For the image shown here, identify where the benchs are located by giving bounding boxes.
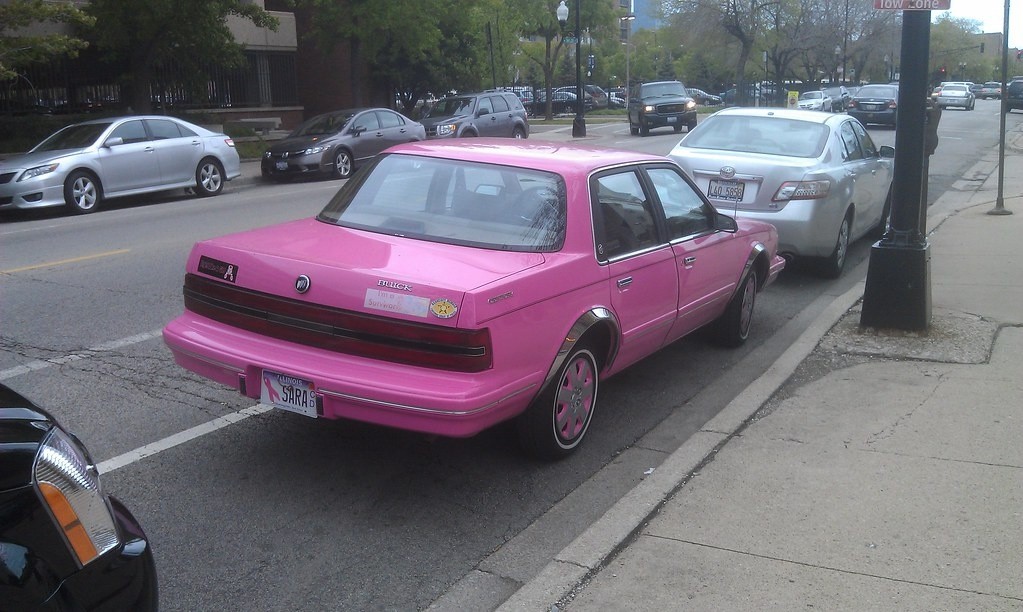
[353,204,557,242]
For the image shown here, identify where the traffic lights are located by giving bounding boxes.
[1017,50,1021,59]
[941,66,946,82]
[980,42,984,53]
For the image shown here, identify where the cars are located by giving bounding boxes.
[664,105,897,280]
[719,81,790,105]
[928,81,984,106]
[937,84,976,111]
[1006,75,1023,91]
[0,383,160,612]
[160,137,786,457]
[0,114,242,218]
[846,80,900,99]
[394,84,628,116]
[846,83,899,127]
[260,107,427,183]
[794,91,833,112]
[821,86,853,112]
[685,88,723,106]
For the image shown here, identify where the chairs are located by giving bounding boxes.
[454,189,500,220]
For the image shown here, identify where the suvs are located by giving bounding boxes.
[1005,80,1023,112]
[628,79,700,137]
[418,92,530,140]
[982,82,1001,100]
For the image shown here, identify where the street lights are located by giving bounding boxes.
[620,11,636,109]
[556,0,588,137]
